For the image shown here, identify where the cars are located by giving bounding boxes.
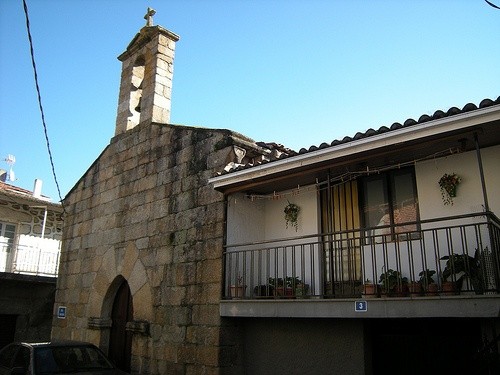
[1,339,127,375]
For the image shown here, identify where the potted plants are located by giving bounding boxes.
[231,275,247,300]
[283,201,300,233]
[362,248,487,296]
[254,275,309,298]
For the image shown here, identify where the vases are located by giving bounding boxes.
[444,185,456,198]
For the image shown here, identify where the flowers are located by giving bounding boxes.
[439,173,462,206]
